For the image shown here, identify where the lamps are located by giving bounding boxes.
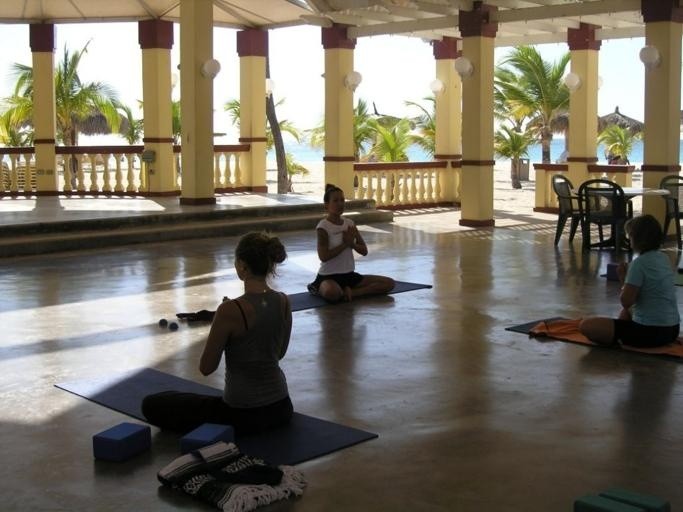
[430,80,444,95]
[639,46,661,71]
[597,74,603,91]
[565,72,581,92]
[344,71,362,92]
[266,78,275,98]
[200,58,221,80]
[454,57,473,77]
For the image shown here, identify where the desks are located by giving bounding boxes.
[576,188,670,256]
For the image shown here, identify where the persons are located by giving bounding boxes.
[140,233,293,441]
[577,215,680,348]
[308,183,395,303]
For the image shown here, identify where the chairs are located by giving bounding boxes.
[551,174,604,249]
[658,174,683,248]
[578,180,633,256]
